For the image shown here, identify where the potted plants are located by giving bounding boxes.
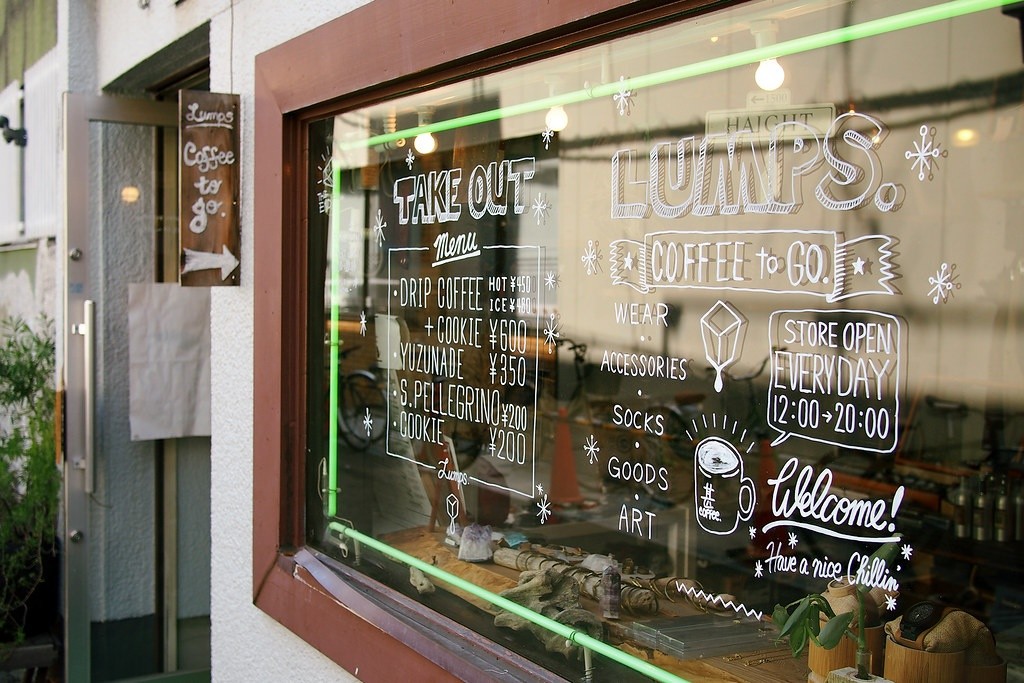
[775,535,895,683]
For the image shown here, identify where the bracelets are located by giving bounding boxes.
[515,540,737,618]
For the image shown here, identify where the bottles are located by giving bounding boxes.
[1012,487,1024,540]
[973,481,992,540]
[602,552,621,619]
[994,475,1011,541]
[954,474,971,537]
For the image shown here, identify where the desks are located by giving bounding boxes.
[504,497,755,580]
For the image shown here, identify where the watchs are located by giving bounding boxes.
[899,595,945,642]
